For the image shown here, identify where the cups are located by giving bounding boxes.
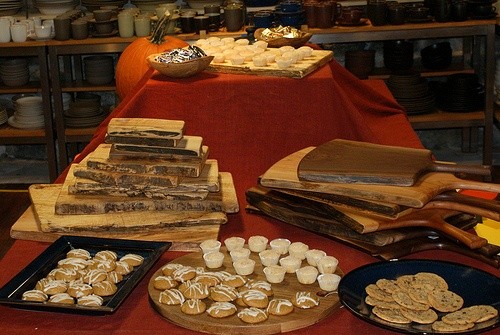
[0,0,498,43]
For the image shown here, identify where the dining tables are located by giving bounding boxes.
[0,43,499,334]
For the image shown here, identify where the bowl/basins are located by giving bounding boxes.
[146,51,216,78]
[383,39,455,75]
[83,55,116,86]
[1,59,31,89]
[254,26,314,47]
[344,49,375,81]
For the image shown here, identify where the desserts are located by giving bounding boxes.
[191,37,313,69]
[200,236,341,291]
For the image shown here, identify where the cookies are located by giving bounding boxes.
[154,264,320,323]
[23,249,144,307]
[365,272,498,332]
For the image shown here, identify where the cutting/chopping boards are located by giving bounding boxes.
[244,139,500,262]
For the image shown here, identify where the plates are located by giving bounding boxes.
[0,92,109,128]
[338,258,500,335]
[386,77,486,115]
[0,234,173,316]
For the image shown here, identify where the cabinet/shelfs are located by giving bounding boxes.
[0,1,500,193]
